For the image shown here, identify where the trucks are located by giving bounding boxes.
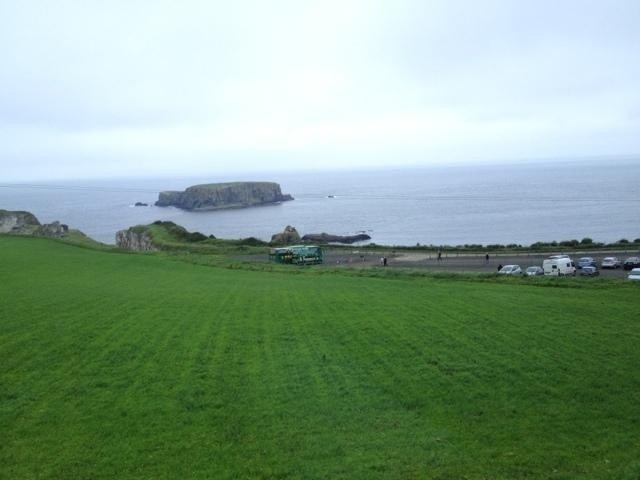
[542,255,577,278]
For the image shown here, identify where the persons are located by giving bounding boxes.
[438,250,442,261]
[498,265,503,271]
[380,257,387,266]
[486,252,489,264]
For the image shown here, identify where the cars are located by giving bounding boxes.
[577,256,640,281]
[497,265,543,276]
